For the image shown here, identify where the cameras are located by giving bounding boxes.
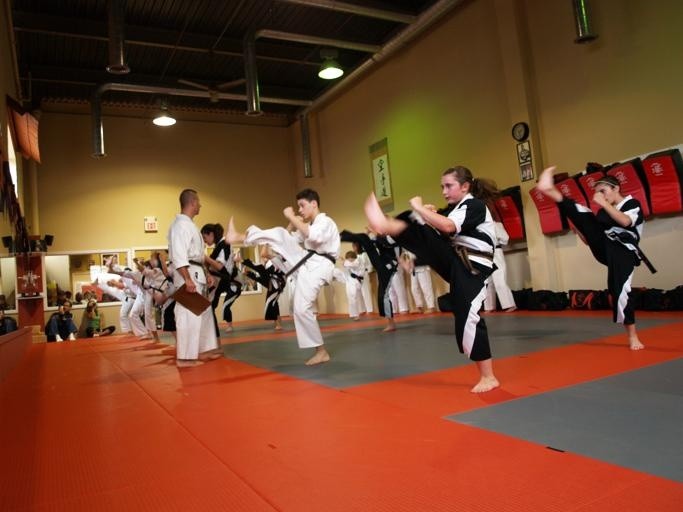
[89,303,96,307]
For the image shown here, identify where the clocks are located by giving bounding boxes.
[511,122,532,144]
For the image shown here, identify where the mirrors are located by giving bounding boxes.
[0,160,36,345]
[40,243,131,312]
[132,244,264,300]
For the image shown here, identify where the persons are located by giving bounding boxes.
[536,165,658,351]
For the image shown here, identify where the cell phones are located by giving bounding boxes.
[59,305,64,314]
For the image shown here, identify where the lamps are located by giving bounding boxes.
[151,95,178,129]
[317,46,347,84]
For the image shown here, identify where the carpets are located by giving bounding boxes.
[200,310,680,393]
[440,354,682,484]
[156,316,390,347]
